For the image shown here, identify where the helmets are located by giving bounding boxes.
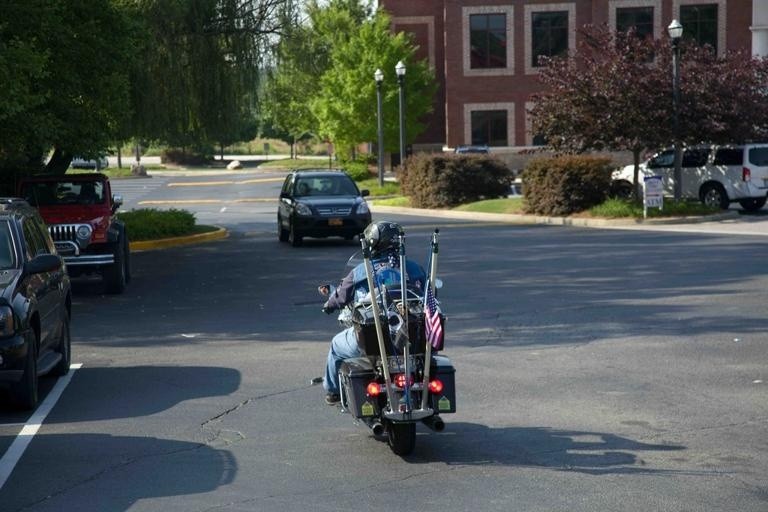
[364,221,405,256]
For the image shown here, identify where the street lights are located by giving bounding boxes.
[373,68,385,188]
[668,19,684,207]
[394,59,407,164]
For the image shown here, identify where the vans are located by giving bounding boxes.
[610,142,767,212]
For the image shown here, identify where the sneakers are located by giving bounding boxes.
[326,392,340,404]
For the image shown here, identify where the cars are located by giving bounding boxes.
[71,151,109,170]
[277,167,371,247]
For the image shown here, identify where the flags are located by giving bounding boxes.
[379,283,405,349]
[422,280,444,351]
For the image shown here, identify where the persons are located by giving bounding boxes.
[321,220,441,408]
[297,182,310,196]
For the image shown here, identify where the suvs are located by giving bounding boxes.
[14,172,132,293]
[0,195,74,411]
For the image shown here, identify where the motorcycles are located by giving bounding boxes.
[308,249,457,461]
